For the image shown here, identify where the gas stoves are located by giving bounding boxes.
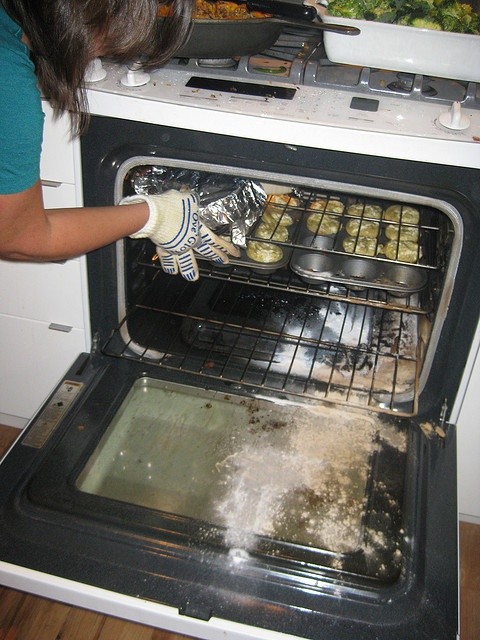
[51,30,479,143]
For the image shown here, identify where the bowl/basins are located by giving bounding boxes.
[320,15,480,82]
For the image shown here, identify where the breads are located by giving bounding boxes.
[346,203,382,220]
[308,200,344,218]
[342,236,379,257]
[269,194,300,209]
[246,242,284,263]
[384,239,422,262]
[304,211,343,235]
[255,221,289,242]
[327,0,480,35]
[260,209,294,227]
[384,222,422,241]
[384,204,420,225]
[345,219,380,237]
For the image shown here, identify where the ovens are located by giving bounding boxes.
[2,93,479,638]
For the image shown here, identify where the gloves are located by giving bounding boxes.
[119,186,242,282]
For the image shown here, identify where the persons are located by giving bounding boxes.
[1,0,243,285]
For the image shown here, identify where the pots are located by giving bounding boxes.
[145,16,358,61]
